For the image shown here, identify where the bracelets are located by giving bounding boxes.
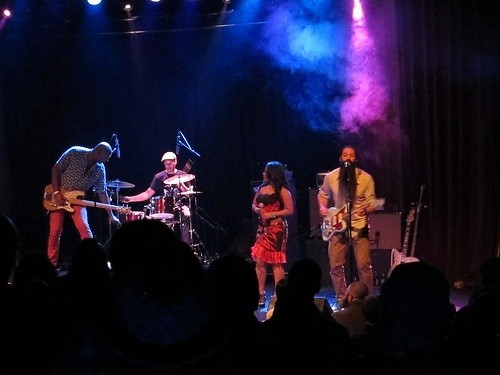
[52,190,59,196]
[271,212,277,218]
[255,208,258,213]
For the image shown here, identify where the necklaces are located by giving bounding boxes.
[267,184,274,195]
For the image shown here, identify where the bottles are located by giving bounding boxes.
[381,272,386,284]
[374,271,379,285]
[284,165,288,178]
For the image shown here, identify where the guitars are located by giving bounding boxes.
[43,184,131,215]
[319,199,385,241]
[387,209,419,279]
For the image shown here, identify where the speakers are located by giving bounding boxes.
[366,211,404,253]
[309,188,335,237]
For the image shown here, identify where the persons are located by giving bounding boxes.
[251,161,295,306]
[0,214,500,375]
[317,146,380,306]
[48,141,121,269]
[123,151,193,244]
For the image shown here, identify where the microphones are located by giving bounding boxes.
[375,231,380,239]
[115,139,120,158]
[175,135,182,154]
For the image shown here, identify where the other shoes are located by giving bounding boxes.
[56,265,61,271]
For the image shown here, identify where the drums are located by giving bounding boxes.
[145,205,179,225]
[151,196,175,220]
[119,211,144,224]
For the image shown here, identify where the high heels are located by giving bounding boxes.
[257,291,267,305]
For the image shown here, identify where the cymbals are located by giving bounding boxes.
[163,174,195,184]
[107,181,135,189]
[177,191,203,198]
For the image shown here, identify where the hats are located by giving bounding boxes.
[161,152,176,162]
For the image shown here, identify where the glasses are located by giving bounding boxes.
[164,162,175,165]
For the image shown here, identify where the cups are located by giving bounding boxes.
[288,171,292,178]
